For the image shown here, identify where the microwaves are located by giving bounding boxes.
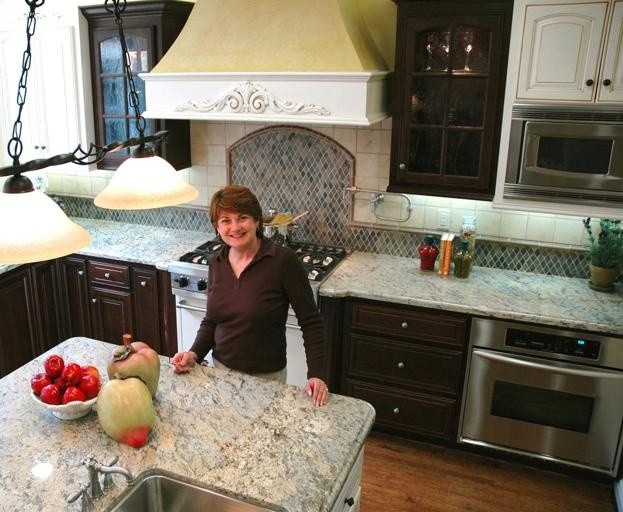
[502,104,623,208]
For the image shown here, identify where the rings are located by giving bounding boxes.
[325,389,328,391]
[320,388,323,391]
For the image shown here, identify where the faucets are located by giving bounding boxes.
[81,452,136,500]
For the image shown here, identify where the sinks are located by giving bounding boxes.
[104,467,287,512]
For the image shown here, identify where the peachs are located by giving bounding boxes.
[97,373,157,449]
[107,333,161,399]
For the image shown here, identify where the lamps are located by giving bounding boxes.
[94,12,198,211]
[1,11,92,265]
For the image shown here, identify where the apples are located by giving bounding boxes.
[31,373,52,395]
[61,363,83,386]
[81,365,99,381]
[41,384,61,404]
[43,355,64,377]
[63,387,85,404]
[80,376,101,398]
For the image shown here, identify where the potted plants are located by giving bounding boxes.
[582,213,623,293]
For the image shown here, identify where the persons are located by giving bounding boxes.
[171,185,329,406]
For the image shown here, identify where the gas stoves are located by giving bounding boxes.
[171,235,345,309]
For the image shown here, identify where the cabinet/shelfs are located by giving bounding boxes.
[55,214,132,343]
[329,443,363,512]
[83,1,194,175]
[511,0,623,109]
[134,221,176,354]
[0,261,54,375]
[321,253,469,452]
[389,0,511,200]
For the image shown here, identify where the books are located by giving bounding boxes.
[439,233,456,276]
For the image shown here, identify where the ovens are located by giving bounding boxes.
[456,316,623,479]
